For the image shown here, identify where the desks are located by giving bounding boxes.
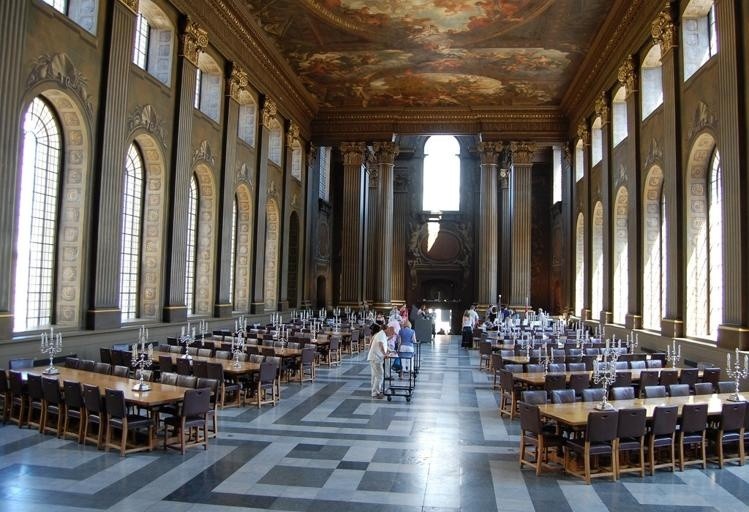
[501,355,651,364]
[489,336,594,344]
[5,365,195,450]
[512,327,552,329]
[232,333,330,367]
[513,367,704,391]
[536,392,749,474]
[124,350,260,407]
[194,339,302,385]
[300,329,352,355]
[492,344,580,353]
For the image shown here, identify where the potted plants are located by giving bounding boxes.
[304,310,305,317]
[582,323,584,331]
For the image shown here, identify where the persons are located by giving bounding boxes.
[469,302,514,322]
[373,303,428,334]
[461,309,474,351]
[397,319,417,381]
[366,325,396,399]
[385,326,399,381]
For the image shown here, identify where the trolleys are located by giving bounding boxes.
[396,339,421,379]
[415,316,433,344]
[383,351,416,403]
[424,314,437,338]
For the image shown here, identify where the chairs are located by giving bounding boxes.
[204,342,220,358]
[114,366,129,378]
[582,389,604,402]
[716,382,736,393]
[113,344,129,351]
[315,331,323,334]
[353,324,360,328]
[170,346,184,354]
[479,342,492,371]
[357,326,371,351]
[292,328,300,336]
[564,343,576,349]
[270,330,278,334]
[122,350,135,371]
[274,341,287,348]
[179,340,187,346]
[695,402,746,469]
[216,350,231,360]
[514,344,527,355]
[317,334,329,340]
[282,342,300,377]
[208,363,241,410]
[679,368,699,394]
[629,361,645,369]
[658,371,678,392]
[261,330,269,334]
[530,343,542,349]
[668,384,689,397]
[159,344,170,352]
[555,339,567,344]
[167,337,178,346]
[580,335,590,338]
[304,332,314,339]
[65,357,79,369]
[247,333,257,338]
[137,352,160,381]
[239,354,265,398]
[246,325,255,333]
[518,350,527,356]
[190,341,204,349]
[658,403,708,472]
[521,390,547,405]
[500,350,514,357]
[618,355,633,361]
[632,354,646,361]
[193,360,208,378]
[611,387,634,400]
[213,335,223,341]
[316,334,329,340]
[213,331,222,335]
[587,326,592,332]
[550,349,565,356]
[221,343,232,351]
[526,364,544,373]
[517,401,568,476]
[234,333,244,338]
[591,373,610,391]
[567,362,586,371]
[584,348,600,355]
[646,359,661,368]
[621,343,631,354]
[281,331,292,336]
[286,349,315,385]
[565,339,576,344]
[155,375,198,437]
[111,349,122,365]
[600,408,647,480]
[484,339,497,344]
[100,347,112,364]
[551,390,576,403]
[267,327,276,331]
[298,338,320,368]
[250,329,261,334]
[515,339,527,344]
[224,352,248,396]
[613,348,627,354]
[189,378,220,443]
[568,374,589,397]
[569,349,582,356]
[544,375,566,399]
[247,346,259,355]
[246,338,258,345]
[188,347,198,356]
[95,363,111,375]
[263,334,273,340]
[79,360,95,372]
[176,358,193,376]
[598,363,610,370]
[222,331,232,336]
[580,343,591,349]
[625,405,679,476]
[234,345,242,352]
[600,348,615,354]
[137,372,178,431]
[565,411,618,485]
[581,356,597,371]
[549,335,560,339]
[473,328,483,347]
[288,337,298,343]
[304,333,316,339]
[83,384,107,450]
[42,377,65,439]
[242,362,277,408]
[492,354,506,391]
[63,380,86,444]
[499,370,521,420]
[732,402,749,462]
[343,330,359,356]
[204,333,211,338]
[602,355,617,362]
[579,339,590,343]
[519,335,527,340]
[126,369,154,414]
[530,349,545,356]
[317,335,341,363]
[610,372,631,387]
[500,339,513,344]
[198,349,212,357]
[694,383,712,395]
[593,339,601,343]
[548,356,565,364]
[530,339,541,344]
[27,373,45,433]
[7,370,29,429]
[584,326,587,331]
[547,343,558,350]
[302,329,310,333]
[159,356,177,377]
[246,357,282,404]
[0,369,11,425]
[548,363,566,372]
[541,339,552,344]
[504,364,523,373]
[698,368,721,394]
[194,334,202,340]
[235,337,245,344]
[224,336,232,342]
[533,336,543,340]
[644,386,665,398]
[480,333,497,344]
[287,343,317,379]
[164,388,210,455]
[9,359,33,370]
[632,371,659,399]
[609,342,617,348]
[529,357,546,364]
[341,324,350,328]
[504,336,514,340]
[651,353,665,368]
[262,348,275,357]
[593,343,606,348]
[256,326,266,330]
[492,326,501,331]
[565,355,581,363]
[317,336,338,367]
[294,332,303,337]
[568,335,576,340]
[294,332,304,337]
[262,340,274,346]
[105,387,155,457]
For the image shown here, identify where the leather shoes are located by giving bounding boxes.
[373,395,384,400]
[384,377,394,381]
[378,394,384,397]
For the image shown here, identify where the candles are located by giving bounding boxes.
[336,307,338,312]
[585,331,588,338]
[203,319,204,326]
[276,313,278,321]
[301,313,303,320]
[206,322,208,331]
[339,318,341,324]
[192,328,193,336]
[139,327,141,339]
[612,334,615,342]
[55,334,58,345]
[678,345,680,354]
[636,334,639,342]
[293,309,295,316]
[518,328,519,335]
[286,329,288,339]
[513,326,515,333]
[352,313,353,319]
[310,323,312,329]
[603,327,605,333]
[59,333,62,343]
[727,354,730,368]
[142,324,144,338]
[338,309,341,313]
[744,355,748,369]
[668,345,669,354]
[244,318,247,329]
[349,315,350,321]
[235,322,237,332]
[355,315,356,321]
[150,343,153,355]
[596,362,599,374]
[322,308,324,314]
[309,309,311,314]
[312,310,314,315]
[237,334,240,347]
[290,312,292,318]
[232,337,234,348]
[606,340,609,349]
[558,320,559,329]
[735,348,738,360]
[595,326,597,334]
[333,317,334,323]
[282,325,284,336]
[345,308,346,312]
[280,316,282,323]
[626,335,629,344]
[242,338,244,349]
[347,306,348,311]
[146,328,148,340]
[553,323,556,332]
[193,327,195,336]
[593,360,596,374]
[324,309,327,315]
[612,362,615,374]
[41,333,44,344]
[610,361,612,375]
[199,321,201,328]
[135,343,137,356]
[148,345,151,357]
[45,332,47,343]
[509,328,512,335]
[318,322,319,329]
[333,308,335,314]
[314,322,315,327]
[319,310,321,316]
[182,327,183,335]
[618,339,621,349]
[349,307,350,312]
[296,311,297,317]
[239,317,241,324]
[604,355,606,365]
[272,316,274,323]
[132,344,135,358]
[188,322,190,333]
[242,314,244,323]
[560,324,564,331]
[576,330,580,338]
[183,327,185,334]
[672,340,675,352]
[307,312,308,318]
[631,331,633,342]
[278,328,280,335]
[50,327,54,338]
[598,324,601,332]
